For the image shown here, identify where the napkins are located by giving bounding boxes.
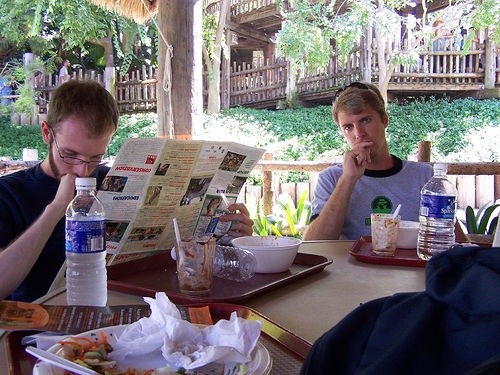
[113,291,265,371]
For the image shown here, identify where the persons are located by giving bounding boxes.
[33,70,42,89]
[0,69,13,107]
[302,80,471,246]
[427,26,488,74]
[58,59,70,86]
[0,80,254,304]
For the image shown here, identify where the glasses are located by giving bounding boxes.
[49,126,110,166]
[333,81,369,103]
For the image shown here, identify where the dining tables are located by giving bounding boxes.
[43,239,424,343]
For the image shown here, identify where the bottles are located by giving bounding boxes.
[170,245,257,282]
[416,164,458,260]
[65,178,108,307]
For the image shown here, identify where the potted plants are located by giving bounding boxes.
[461,202,500,244]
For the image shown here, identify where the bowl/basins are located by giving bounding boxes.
[396,220,420,249]
[231,235,302,274]
[32,323,273,375]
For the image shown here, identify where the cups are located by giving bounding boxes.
[175,235,216,295]
[371,213,401,256]
